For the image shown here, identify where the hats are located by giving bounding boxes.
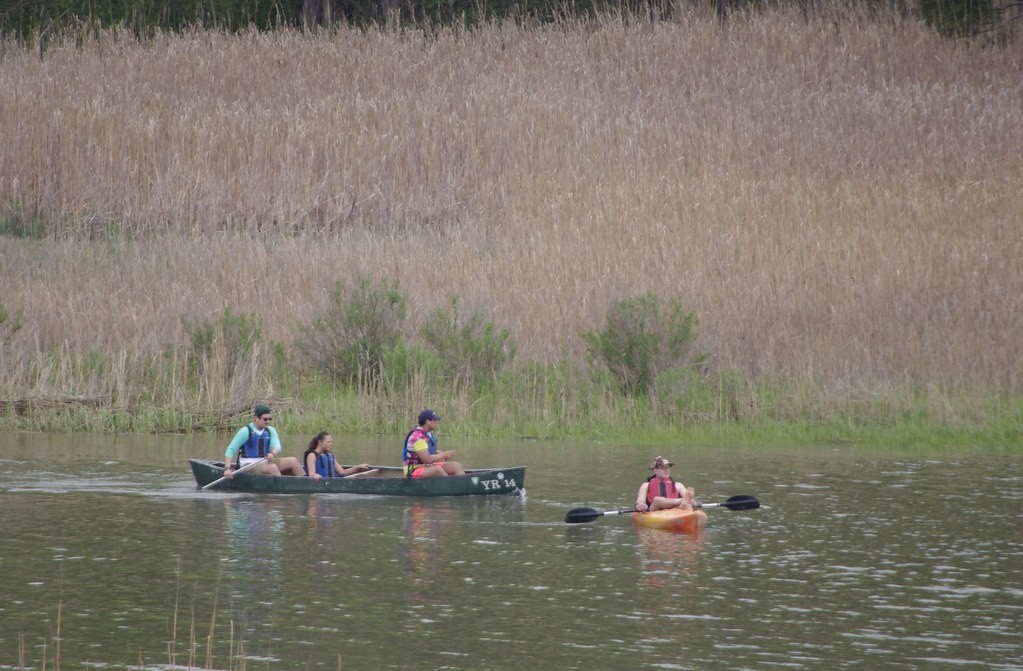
[650,456,674,470]
[255,406,270,415]
[420,409,441,421]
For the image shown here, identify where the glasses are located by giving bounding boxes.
[264,418,271,421]
[654,465,670,470]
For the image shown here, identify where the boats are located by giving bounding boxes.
[189,457,528,497]
[631,509,709,537]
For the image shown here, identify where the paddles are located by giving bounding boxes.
[564,494,760,523]
[195,456,268,490]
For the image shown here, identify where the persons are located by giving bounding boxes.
[224,405,306,481]
[636,456,696,510]
[402,409,464,477]
[303,431,370,479]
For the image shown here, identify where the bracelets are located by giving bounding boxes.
[271,452,275,457]
